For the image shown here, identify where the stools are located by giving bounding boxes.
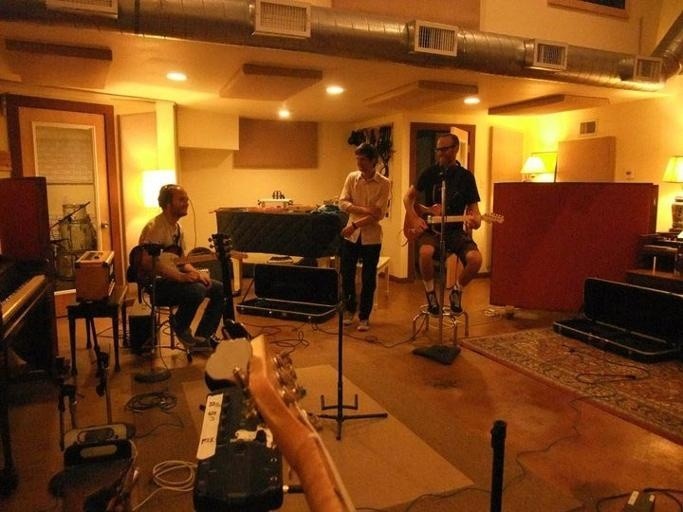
[154,305,192,363]
[356,257,390,308]
[413,249,468,346]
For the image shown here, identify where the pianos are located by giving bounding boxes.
[1,259,55,349]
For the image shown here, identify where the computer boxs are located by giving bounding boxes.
[128,296,160,354]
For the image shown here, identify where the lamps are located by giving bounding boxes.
[662,156,683,232]
[520,154,547,182]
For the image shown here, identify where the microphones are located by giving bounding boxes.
[439,157,446,177]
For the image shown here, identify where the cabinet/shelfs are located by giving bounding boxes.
[624,233,682,295]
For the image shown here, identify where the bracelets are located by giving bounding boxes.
[352,221,358,230]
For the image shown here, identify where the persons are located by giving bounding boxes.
[137,184,225,352]
[337,142,392,331]
[403,132,483,316]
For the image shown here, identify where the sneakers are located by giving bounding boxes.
[425,289,440,317]
[189,335,219,353]
[448,287,464,315]
[356,319,370,331]
[167,314,196,346]
[342,311,353,326]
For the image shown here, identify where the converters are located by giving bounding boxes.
[625,489,656,512]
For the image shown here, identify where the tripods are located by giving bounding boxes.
[305,300,387,439]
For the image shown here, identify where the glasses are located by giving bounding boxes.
[433,145,458,153]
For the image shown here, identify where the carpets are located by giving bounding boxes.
[461,326,682,445]
[182,364,474,510]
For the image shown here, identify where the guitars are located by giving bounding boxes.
[128,243,248,281]
[210,234,251,341]
[403,202,504,234]
[234,334,357,507]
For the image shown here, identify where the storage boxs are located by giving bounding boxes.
[73,250,116,303]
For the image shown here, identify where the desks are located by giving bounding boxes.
[65,285,129,371]
[215,208,348,292]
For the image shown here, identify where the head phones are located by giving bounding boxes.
[163,185,172,204]
